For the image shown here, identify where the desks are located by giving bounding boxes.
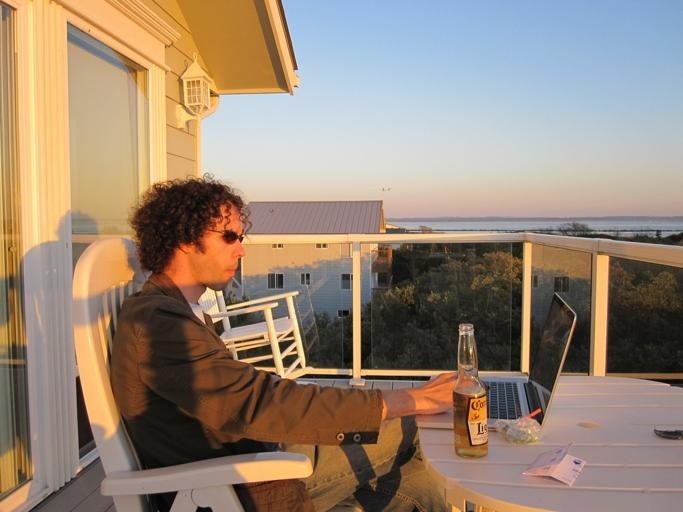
[416,372,682,512]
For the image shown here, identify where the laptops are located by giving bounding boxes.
[414,293,577,431]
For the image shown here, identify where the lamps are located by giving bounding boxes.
[179,52,215,117]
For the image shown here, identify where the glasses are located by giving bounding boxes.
[208,229,243,245]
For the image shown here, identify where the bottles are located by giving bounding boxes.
[452,324,488,458]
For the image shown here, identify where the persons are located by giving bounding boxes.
[110,176,490,512]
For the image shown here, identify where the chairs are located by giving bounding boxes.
[70,232,314,512]
